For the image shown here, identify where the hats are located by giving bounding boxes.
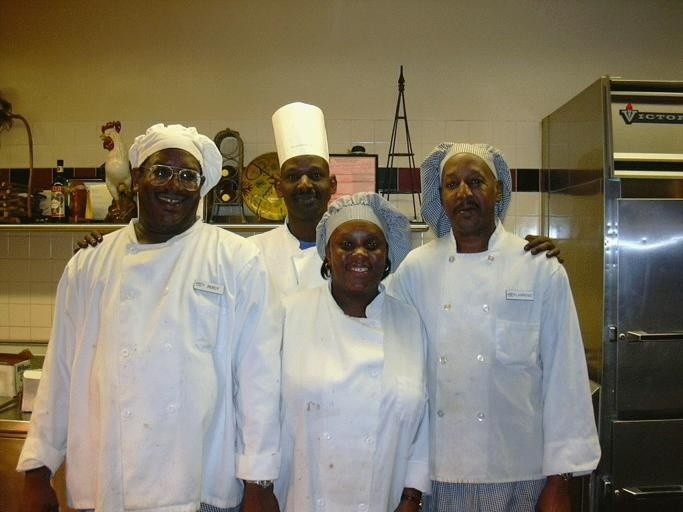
[271,102,330,167]
[128,123,222,196]
[420,141,512,239]
[316,192,413,273]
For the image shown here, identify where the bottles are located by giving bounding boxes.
[50,160,68,223]
[222,165,235,178]
[219,190,237,202]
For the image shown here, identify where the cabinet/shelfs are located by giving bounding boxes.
[0,420,67,512]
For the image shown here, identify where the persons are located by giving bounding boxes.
[387,140,603,512]
[16,123,278,512]
[69,101,564,512]
[268,192,433,512]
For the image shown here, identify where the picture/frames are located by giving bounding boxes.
[329,154,378,193]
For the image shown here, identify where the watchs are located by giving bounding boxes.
[400,494,425,508]
[556,472,575,481]
[245,479,275,489]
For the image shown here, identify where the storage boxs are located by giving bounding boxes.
[22,369,43,412]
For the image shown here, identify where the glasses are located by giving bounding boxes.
[148,165,201,192]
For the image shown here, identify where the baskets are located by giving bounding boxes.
[0,108,46,226]
